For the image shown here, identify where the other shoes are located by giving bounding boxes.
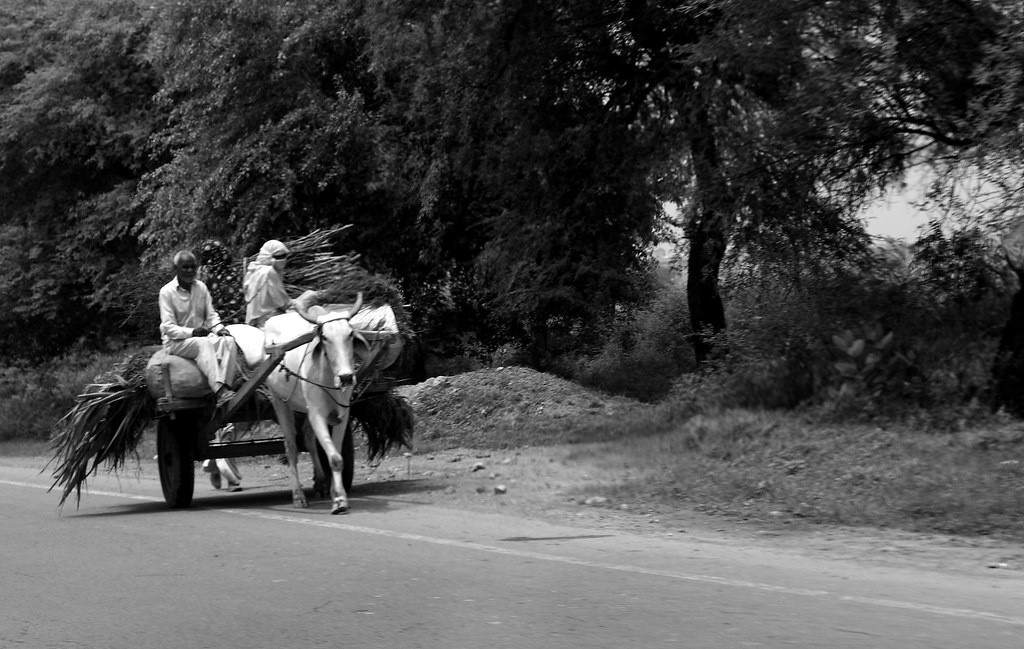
[216,392,236,408]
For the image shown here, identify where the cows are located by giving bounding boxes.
[265,291,372,515]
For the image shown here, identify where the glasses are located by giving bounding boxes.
[182,264,198,269]
[273,253,287,259]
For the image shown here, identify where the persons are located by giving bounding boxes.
[160,251,243,491]
[244,241,296,328]
